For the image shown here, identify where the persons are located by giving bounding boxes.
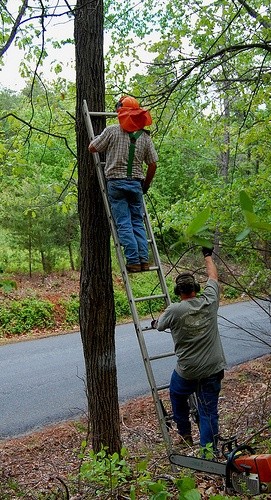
[89,95,159,272]
[151,246,227,459]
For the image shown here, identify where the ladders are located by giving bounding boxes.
[79,97,219,486]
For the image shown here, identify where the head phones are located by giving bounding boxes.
[115,97,126,110]
[174,273,200,295]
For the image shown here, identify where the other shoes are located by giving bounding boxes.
[126,263,149,272]
[174,435,193,447]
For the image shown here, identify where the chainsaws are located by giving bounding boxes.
[167,448,271,496]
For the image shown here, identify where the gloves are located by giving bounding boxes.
[202,246,213,256]
[151,320,156,328]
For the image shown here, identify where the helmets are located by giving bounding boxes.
[116,95,140,108]
